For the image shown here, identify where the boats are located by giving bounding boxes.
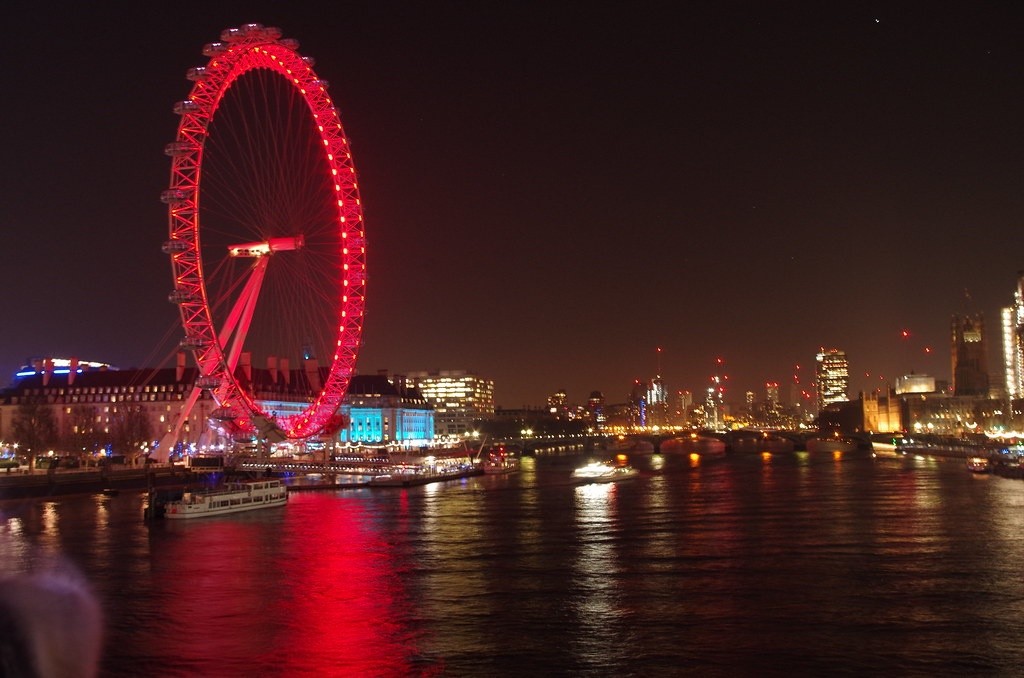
[161,478,290,518]
[484,447,519,474]
